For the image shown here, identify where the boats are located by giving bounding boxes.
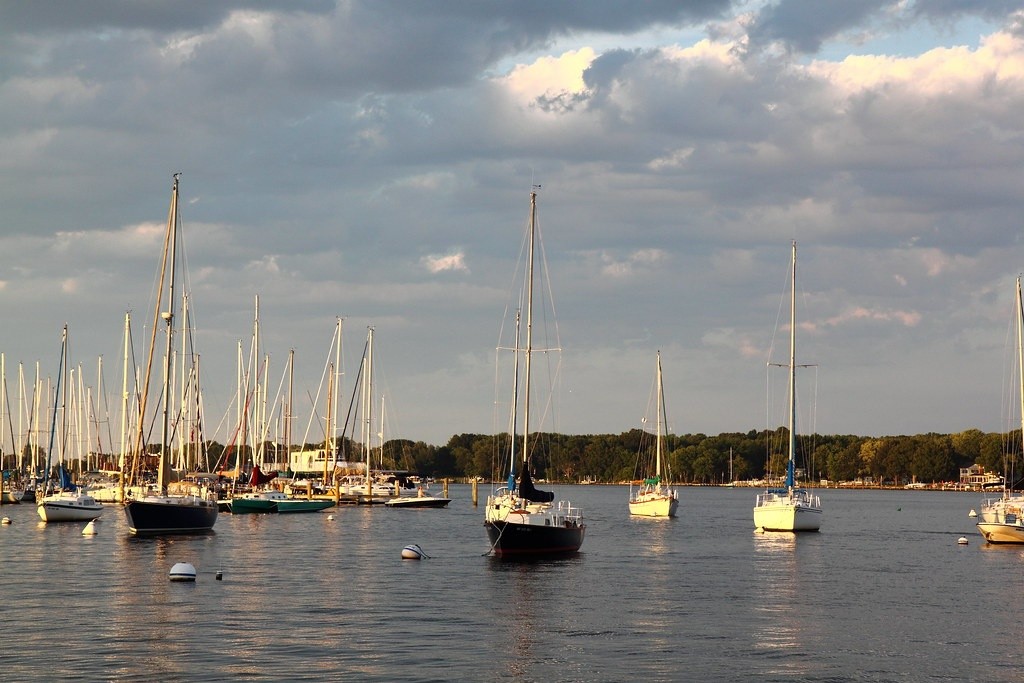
[630,349,679,519]
[483,170,587,562]
[974,276,1024,544]
[753,238,823,532]
[0,169,455,536]
